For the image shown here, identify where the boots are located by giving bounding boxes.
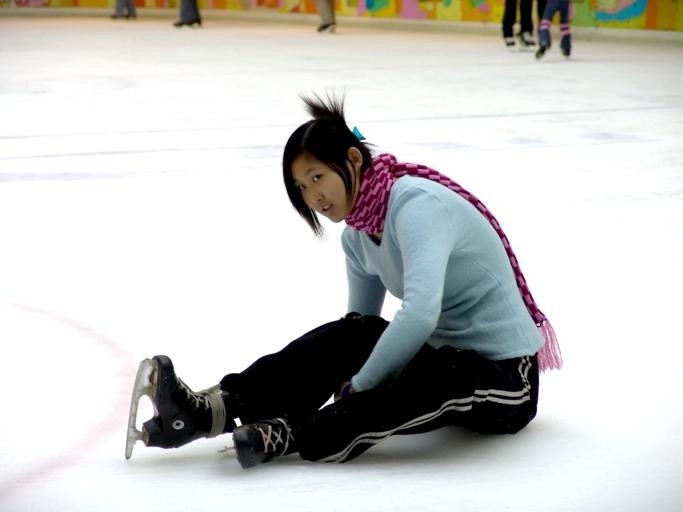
[233,416,299,468]
[141,355,236,448]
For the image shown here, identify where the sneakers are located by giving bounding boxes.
[536,45,569,57]
[174,17,200,25]
[112,14,135,18]
[318,21,334,32]
[505,32,535,45]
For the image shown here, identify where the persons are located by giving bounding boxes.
[531,1,571,59]
[139,90,563,471]
[312,1,337,34]
[171,0,202,27]
[109,1,137,19]
[502,1,537,48]
